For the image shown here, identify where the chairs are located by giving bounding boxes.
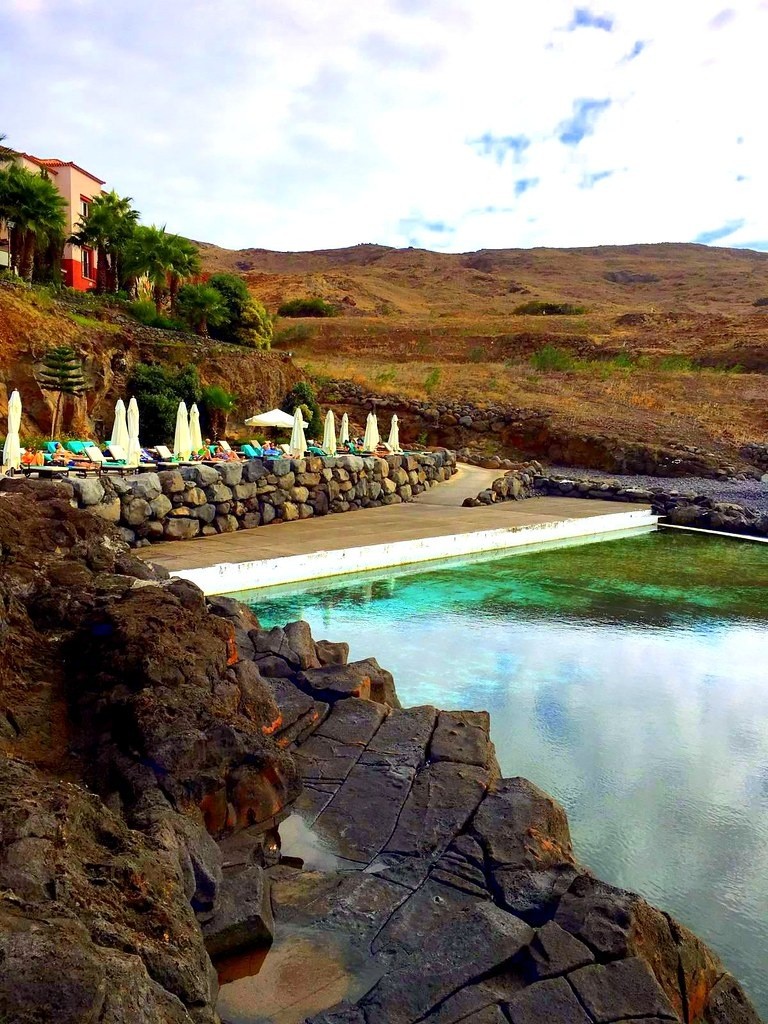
[43,441,102,478]
[65,438,391,479]
[14,447,69,481]
[44,454,87,481]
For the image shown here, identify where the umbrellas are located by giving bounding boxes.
[174,402,190,460]
[127,396,140,466]
[189,404,202,452]
[339,412,349,444]
[111,399,129,450]
[364,412,379,451]
[290,408,307,457]
[3,388,22,477]
[322,410,336,455]
[388,414,399,450]
[245,409,308,446]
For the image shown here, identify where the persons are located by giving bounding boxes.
[54,443,86,458]
[22,446,44,466]
[344,437,362,452]
[189,451,211,461]
[199,439,211,454]
[263,443,294,459]
[313,439,322,448]
[51,453,94,466]
[214,447,245,462]
